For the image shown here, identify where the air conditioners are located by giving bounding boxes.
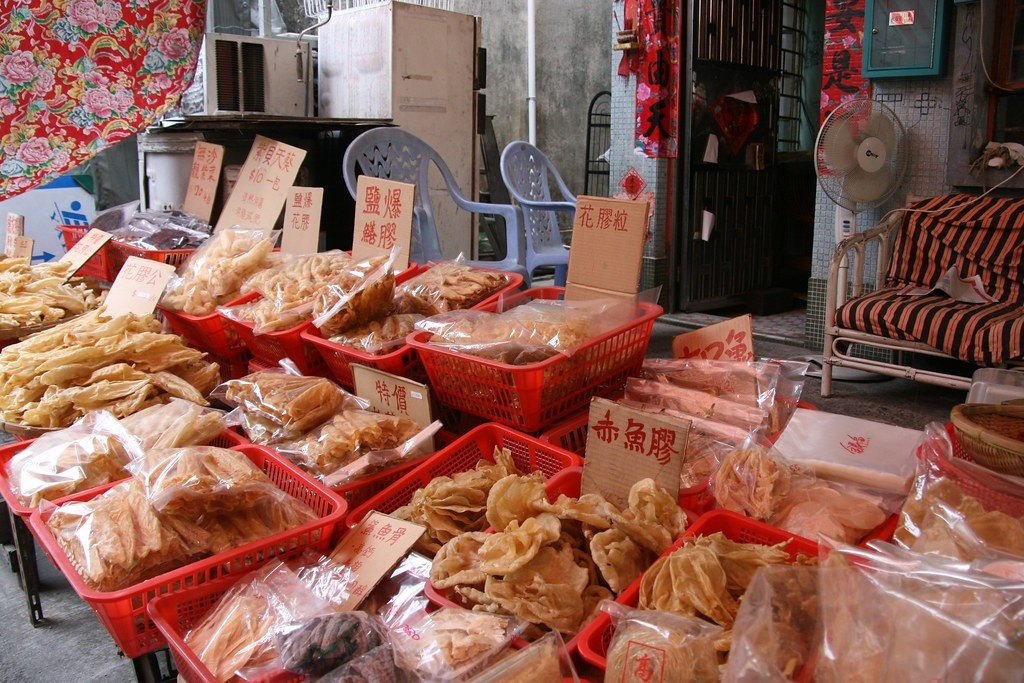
[161,37,313,122]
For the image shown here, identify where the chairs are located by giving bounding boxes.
[342,127,531,290]
[499,141,589,299]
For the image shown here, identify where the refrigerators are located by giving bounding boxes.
[317,0,486,263]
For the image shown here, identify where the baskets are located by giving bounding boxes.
[0,225,1024,680]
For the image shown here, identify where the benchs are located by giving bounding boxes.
[821,194,1024,398]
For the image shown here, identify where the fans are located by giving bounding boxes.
[788,99,908,381]
